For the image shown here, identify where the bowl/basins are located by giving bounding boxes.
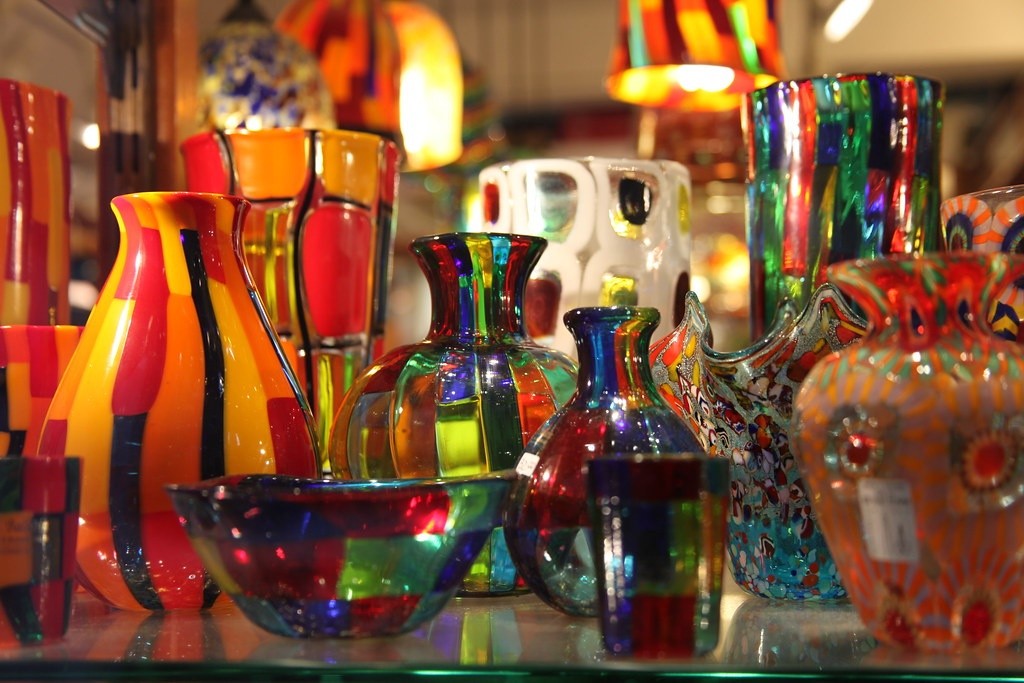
[167,467,523,640]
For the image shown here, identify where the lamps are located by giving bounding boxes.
[270,0,463,174]
[602,0,784,113]
[195,0,336,133]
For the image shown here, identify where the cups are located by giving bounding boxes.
[0,453,85,646]
[585,448,729,661]
